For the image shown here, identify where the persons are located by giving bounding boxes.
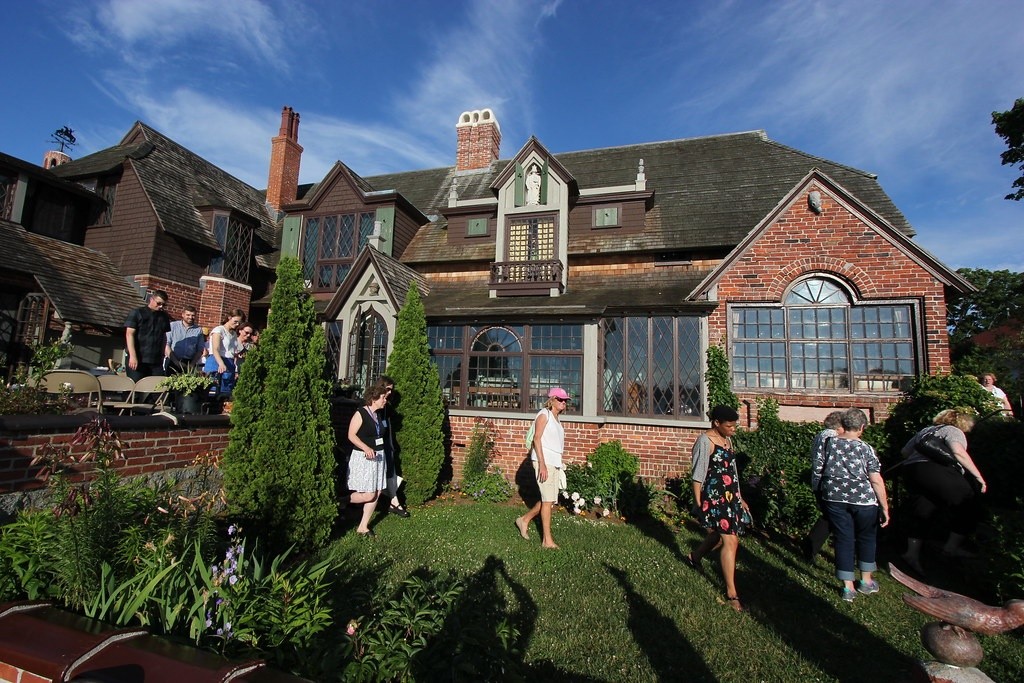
[204,309,246,395]
[981,373,1014,417]
[377,376,409,517]
[337,385,387,538]
[124,290,171,383]
[819,408,890,602]
[801,411,845,562]
[234,322,252,375]
[687,405,749,611]
[194,327,209,371]
[900,407,987,571]
[515,387,571,549]
[250,330,260,345]
[162,305,205,377]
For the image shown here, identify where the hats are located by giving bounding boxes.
[548,388,570,400]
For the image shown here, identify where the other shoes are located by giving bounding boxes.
[516,518,530,540]
[541,543,559,549]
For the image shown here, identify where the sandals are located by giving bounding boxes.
[388,504,408,516]
[726,595,744,613]
[686,552,705,571]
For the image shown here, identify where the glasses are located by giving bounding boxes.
[555,397,567,403]
[152,297,165,307]
[243,329,254,336]
[387,388,393,392]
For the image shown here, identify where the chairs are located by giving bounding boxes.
[37,369,176,416]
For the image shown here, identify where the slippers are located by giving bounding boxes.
[365,530,376,539]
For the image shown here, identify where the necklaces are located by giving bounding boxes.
[712,429,726,447]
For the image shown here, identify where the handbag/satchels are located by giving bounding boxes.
[914,423,958,466]
[382,474,403,496]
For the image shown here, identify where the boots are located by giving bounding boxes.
[943,532,969,554]
[901,537,922,575]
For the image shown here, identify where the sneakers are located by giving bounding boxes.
[841,588,857,603]
[857,579,880,594]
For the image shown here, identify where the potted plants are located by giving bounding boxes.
[154,358,218,413]
